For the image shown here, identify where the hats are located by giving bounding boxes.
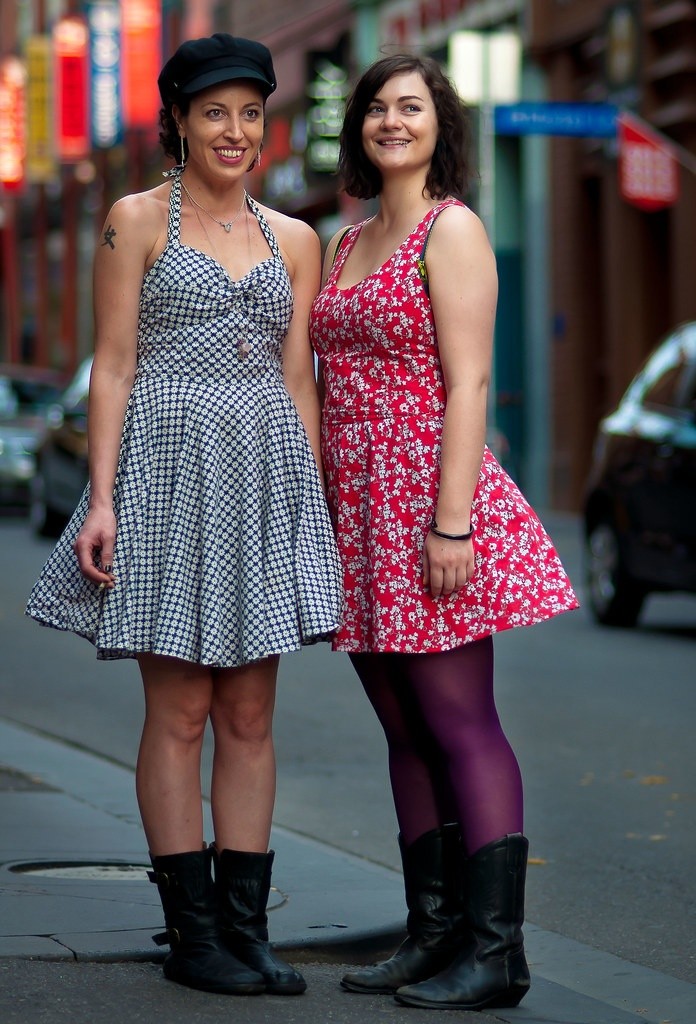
[157,33,277,113]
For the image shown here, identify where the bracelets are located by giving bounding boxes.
[430,520,474,540]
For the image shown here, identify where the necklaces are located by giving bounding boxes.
[180,181,246,233]
[185,190,251,256]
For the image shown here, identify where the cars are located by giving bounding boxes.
[28,354,93,537]
[0,361,75,515]
[576,320,696,626]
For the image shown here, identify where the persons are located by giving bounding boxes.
[309,53,579,1010]
[26,33,342,997]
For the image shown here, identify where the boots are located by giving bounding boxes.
[394,832,531,1009]
[340,823,467,994]
[210,841,308,996]
[146,841,266,995]
